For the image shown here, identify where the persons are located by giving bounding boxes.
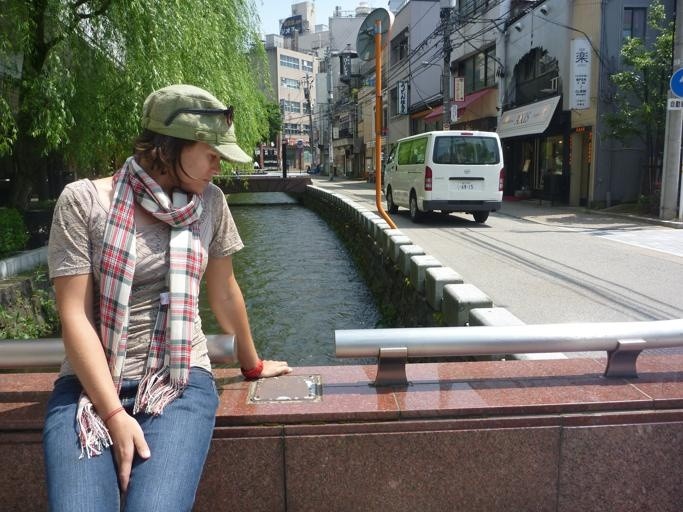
[42,82,291,511]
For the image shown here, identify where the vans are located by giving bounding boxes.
[382,131,504,225]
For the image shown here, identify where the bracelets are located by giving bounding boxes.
[238,361,265,379]
[98,404,123,422]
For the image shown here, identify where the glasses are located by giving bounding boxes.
[165,105,234,126]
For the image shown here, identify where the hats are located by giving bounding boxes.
[140,84,254,164]
